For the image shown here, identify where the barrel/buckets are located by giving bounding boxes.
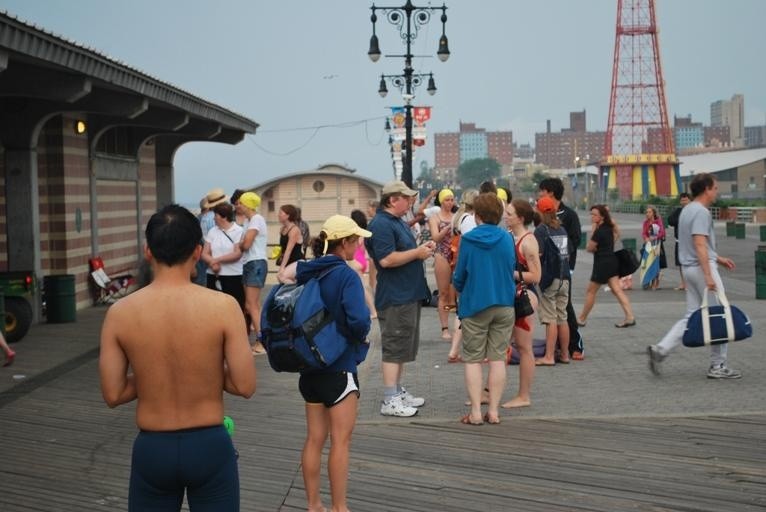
[578,233,586,249]
[44,275,77,323]
[662,215,669,229]
[725,221,766,301]
[623,238,637,255]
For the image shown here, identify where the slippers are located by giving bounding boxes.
[483,412,500,425]
[446,352,464,363]
[460,412,485,427]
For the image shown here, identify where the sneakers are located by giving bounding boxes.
[2,352,18,367]
[399,385,425,408]
[705,364,743,380]
[645,344,667,378]
[613,319,637,329]
[380,394,419,418]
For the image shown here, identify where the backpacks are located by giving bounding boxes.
[257,261,351,375]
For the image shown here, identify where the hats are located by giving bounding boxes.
[271,245,283,260]
[319,214,373,241]
[536,197,555,213]
[438,188,454,203]
[203,188,229,209]
[239,191,263,210]
[381,180,420,198]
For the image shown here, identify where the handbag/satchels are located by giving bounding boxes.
[682,287,753,349]
[514,289,535,319]
[612,247,642,280]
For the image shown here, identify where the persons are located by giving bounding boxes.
[643,172,743,380]
[296,212,371,512]
[96,203,256,511]
[362,180,438,418]
[464,199,541,409]
[452,190,517,426]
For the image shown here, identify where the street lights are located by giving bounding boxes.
[574,155,582,206]
[368,3,451,195]
[584,153,591,209]
[688,168,694,181]
[602,172,609,206]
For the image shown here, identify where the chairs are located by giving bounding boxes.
[88,255,134,306]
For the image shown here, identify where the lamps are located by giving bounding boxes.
[73,120,85,135]
